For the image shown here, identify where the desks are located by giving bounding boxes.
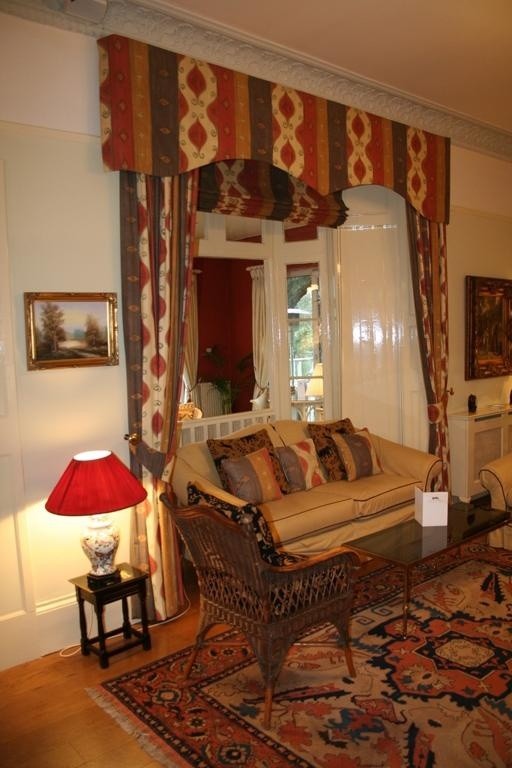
[340,500,512,636]
[290,394,324,421]
[67,561,153,667]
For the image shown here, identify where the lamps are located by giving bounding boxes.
[44,450,149,583]
[305,363,324,420]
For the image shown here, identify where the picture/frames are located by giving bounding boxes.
[23,291,122,374]
[461,274,512,380]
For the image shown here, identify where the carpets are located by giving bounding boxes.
[99,537,512,767]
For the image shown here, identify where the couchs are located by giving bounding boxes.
[162,418,446,595]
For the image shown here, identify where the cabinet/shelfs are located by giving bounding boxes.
[448,402,511,501]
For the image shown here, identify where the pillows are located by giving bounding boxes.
[203,418,384,503]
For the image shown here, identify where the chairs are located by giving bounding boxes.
[478,449,512,553]
[159,491,361,729]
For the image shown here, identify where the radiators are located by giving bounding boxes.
[190,378,234,418]
[178,406,276,448]
